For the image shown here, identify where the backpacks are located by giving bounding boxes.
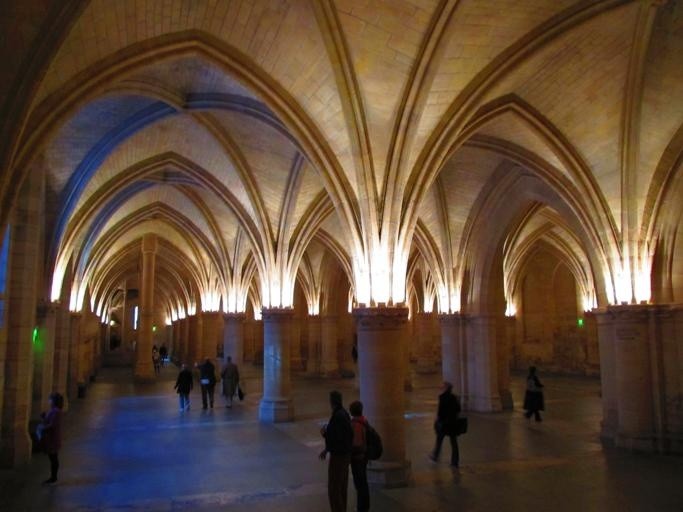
[352,419,383,461]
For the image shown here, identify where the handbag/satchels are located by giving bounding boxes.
[238,388,245,402]
[456,416,468,435]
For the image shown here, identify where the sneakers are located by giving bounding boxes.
[524,412,543,422]
[450,460,459,469]
[428,453,438,461]
[42,478,57,485]
[180,401,232,414]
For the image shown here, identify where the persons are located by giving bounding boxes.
[521,365,546,422]
[152,344,158,350]
[152,351,161,377]
[428,380,464,469]
[195,356,216,410]
[160,341,167,362]
[35,392,65,484]
[348,399,372,512]
[172,363,194,413]
[219,355,240,409]
[317,389,353,512]
